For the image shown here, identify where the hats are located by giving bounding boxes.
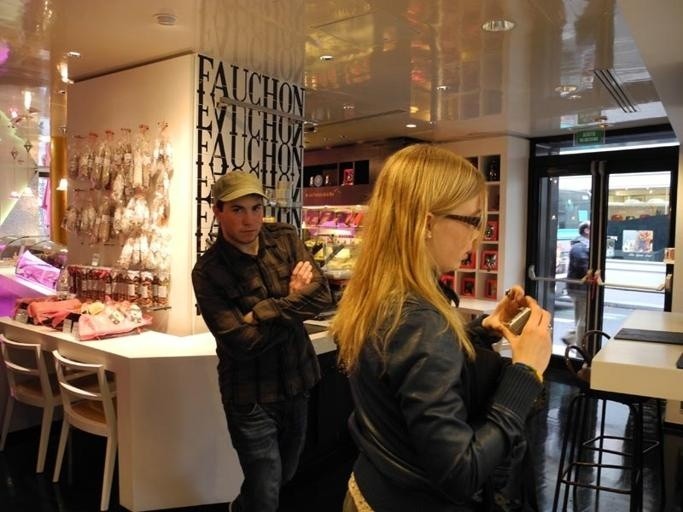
[212,171,270,206]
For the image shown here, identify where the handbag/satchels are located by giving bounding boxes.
[27,298,82,326]
[78,303,153,341]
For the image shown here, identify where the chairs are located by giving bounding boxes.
[0,334,117,511]
[550,329,669,512]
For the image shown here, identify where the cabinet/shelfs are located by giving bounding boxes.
[438,137,528,303]
[298,205,369,263]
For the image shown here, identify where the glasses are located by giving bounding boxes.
[438,213,482,232]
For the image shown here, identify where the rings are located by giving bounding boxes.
[549,324,552,333]
[505,289,513,299]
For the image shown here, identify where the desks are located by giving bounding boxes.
[588,308,683,401]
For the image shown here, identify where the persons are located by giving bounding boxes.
[561,221,590,359]
[191,170,333,512]
[328,143,552,512]
